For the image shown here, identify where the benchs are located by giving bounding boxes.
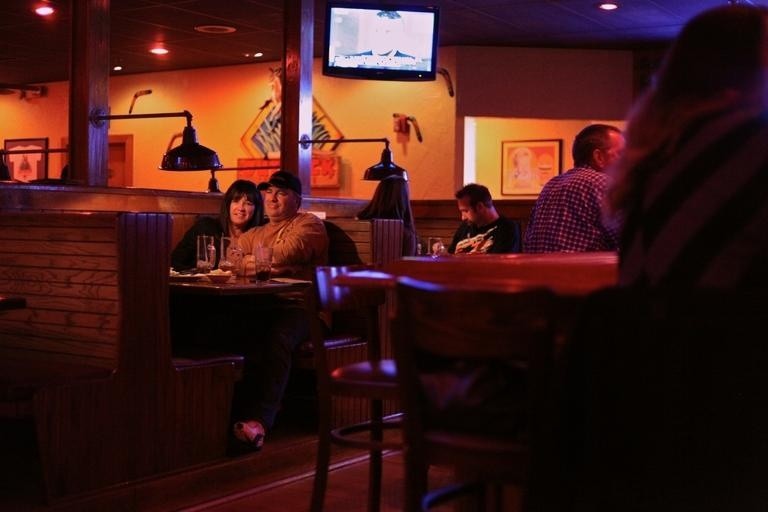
[130,209,247,479]
[292,219,380,436]
[411,216,525,255]
[0,213,128,506]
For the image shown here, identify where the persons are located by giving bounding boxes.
[171,178,265,272]
[448,181,522,252]
[608,2,767,511]
[225,171,333,451]
[329,9,417,69]
[355,172,417,258]
[528,118,625,253]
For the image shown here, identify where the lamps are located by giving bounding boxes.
[88,104,224,172]
[299,133,409,182]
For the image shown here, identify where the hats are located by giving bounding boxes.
[257,172,301,194]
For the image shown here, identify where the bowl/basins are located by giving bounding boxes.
[206,272,233,284]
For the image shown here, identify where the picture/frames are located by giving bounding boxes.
[499,137,563,197]
[2,136,49,181]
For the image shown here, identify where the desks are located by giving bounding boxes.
[168,275,313,298]
[396,248,619,299]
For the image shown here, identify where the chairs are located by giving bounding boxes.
[305,262,405,512]
[388,274,561,512]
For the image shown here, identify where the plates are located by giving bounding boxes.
[170,276,203,281]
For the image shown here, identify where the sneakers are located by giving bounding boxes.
[235,420,265,447]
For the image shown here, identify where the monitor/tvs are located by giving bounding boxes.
[321,0,440,82]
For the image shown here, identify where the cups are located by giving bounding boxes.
[254,245,273,281]
[194,235,216,274]
[220,236,242,271]
